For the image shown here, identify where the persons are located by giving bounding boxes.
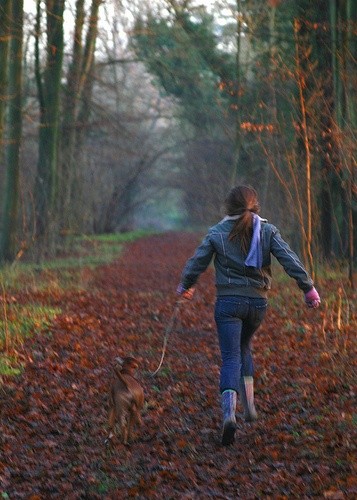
[174,184,321,447]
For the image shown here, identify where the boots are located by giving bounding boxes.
[238,375,258,422]
[219,389,237,446]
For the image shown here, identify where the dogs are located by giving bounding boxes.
[105,357,145,447]
